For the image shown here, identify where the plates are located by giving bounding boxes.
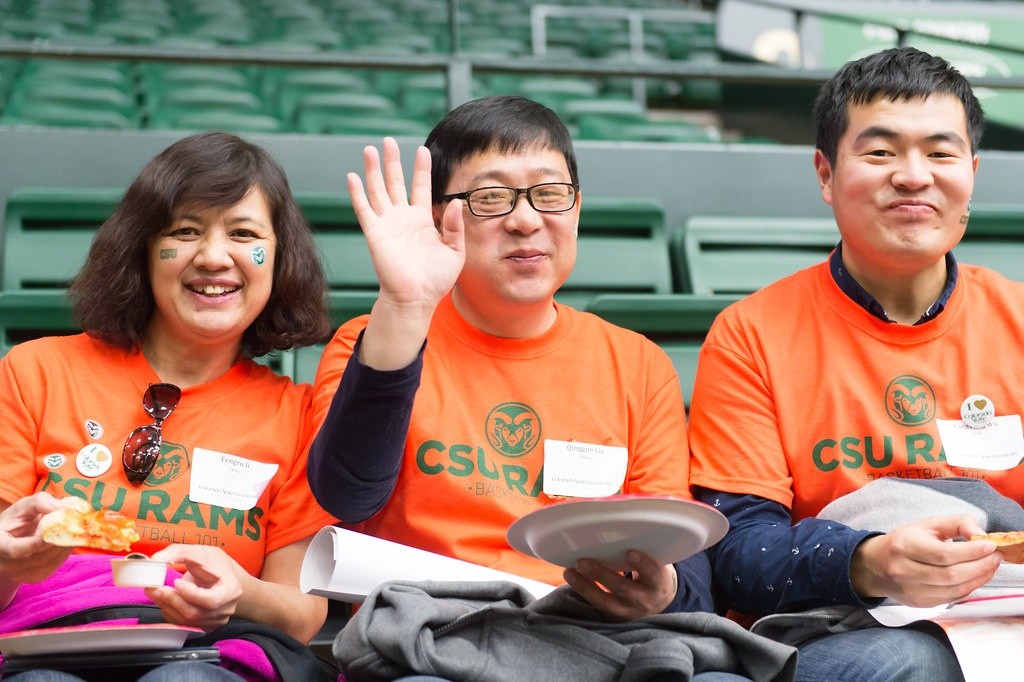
[0,626,206,658]
[507,497,730,574]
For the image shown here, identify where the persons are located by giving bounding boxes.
[0,133,343,682]
[688,47,1024,682]
[302,97,752,682]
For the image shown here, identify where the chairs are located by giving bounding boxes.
[0,0,1024,416]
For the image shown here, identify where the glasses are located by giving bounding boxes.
[122,382,181,489]
[437,182,580,218]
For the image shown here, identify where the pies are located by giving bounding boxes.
[38,506,139,550]
[971,531,1024,565]
